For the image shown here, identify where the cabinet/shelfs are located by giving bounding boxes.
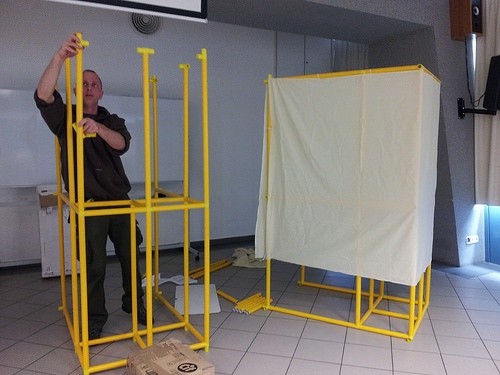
[261,62,443,342]
[49,32,210,375]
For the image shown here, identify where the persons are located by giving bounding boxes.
[32,32,154,348]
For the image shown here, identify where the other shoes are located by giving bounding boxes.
[122,299,147,324]
[89,311,108,340]
